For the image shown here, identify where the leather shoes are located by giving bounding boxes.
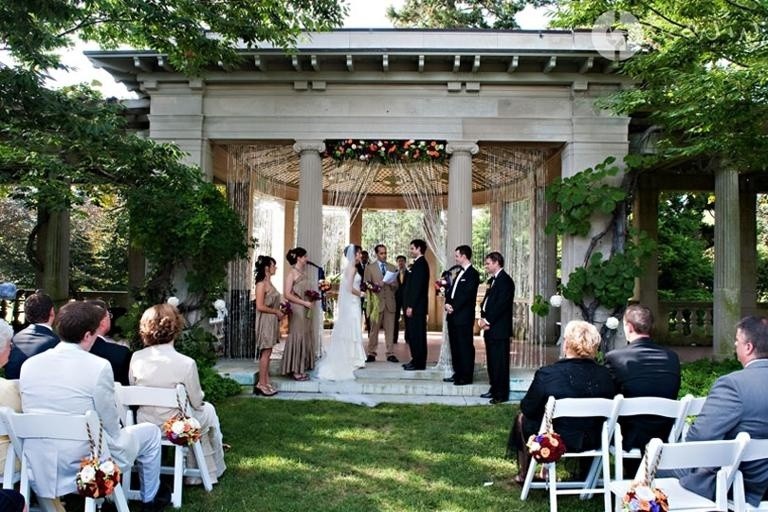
[443,375,462,382]
[490,396,509,404]
[387,355,399,363]
[365,354,376,363]
[404,364,426,371]
[480,391,499,398]
[454,379,474,386]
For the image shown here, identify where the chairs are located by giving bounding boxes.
[3,407,134,511]
[112,381,214,508]
[0,408,22,489]
[519,393,767,512]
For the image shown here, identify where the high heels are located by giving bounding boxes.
[255,382,275,396]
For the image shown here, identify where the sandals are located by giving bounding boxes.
[289,372,306,382]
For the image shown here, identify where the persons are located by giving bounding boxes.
[280,246,315,381]
[654,315,768,508]
[443,245,480,386]
[505,320,616,483]
[479,252,515,404]
[253,255,284,396]
[605,305,681,478]
[314,239,431,381]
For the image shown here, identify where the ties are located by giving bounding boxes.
[380,263,386,278]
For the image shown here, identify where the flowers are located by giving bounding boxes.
[161,414,202,446]
[73,458,126,498]
[278,300,292,317]
[318,279,332,293]
[303,289,321,318]
[360,280,382,317]
[433,279,447,299]
